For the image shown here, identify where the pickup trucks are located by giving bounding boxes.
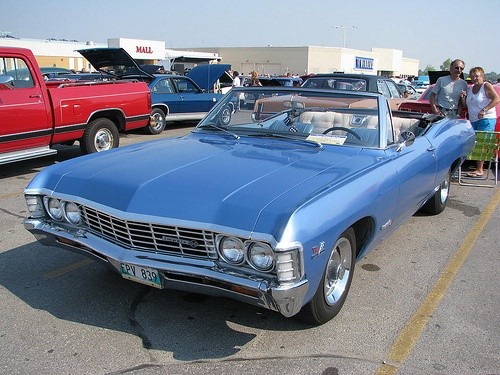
[0,46,152,165]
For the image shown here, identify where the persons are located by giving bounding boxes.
[429,60,467,118]
[248,71,263,103]
[281,72,302,88]
[403,91,410,98]
[461,67,500,177]
[160,69,164,73]
[231,71,241,111]
[411,75,416,82]
[81,68,85,72]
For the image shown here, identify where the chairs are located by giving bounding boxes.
[269,120,314,134]
[345,127,393,148]
[452,130,500,188]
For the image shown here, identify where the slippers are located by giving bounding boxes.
[467,172,484,177]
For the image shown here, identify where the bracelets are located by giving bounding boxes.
[482,108,487,111]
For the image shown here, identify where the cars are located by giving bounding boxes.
[23,86,477,325]
[0,47,500,159]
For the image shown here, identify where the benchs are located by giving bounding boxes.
[298,111,419,143]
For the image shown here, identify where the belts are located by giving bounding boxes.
[440,107,457,111]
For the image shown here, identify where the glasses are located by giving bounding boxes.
[453,65,464,70]
